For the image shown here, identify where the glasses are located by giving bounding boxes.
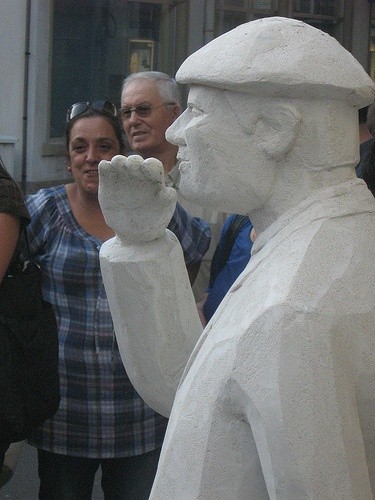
[66,100,117,123]
[117,102,177,120]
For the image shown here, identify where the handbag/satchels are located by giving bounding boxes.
[0,170,62,442]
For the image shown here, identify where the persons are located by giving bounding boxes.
[355,68,375,198]
[115,69,187,193]
[195,211,256,331]
[98,14,375,499]
[0,141,33,500]
[17,99,210,500]
